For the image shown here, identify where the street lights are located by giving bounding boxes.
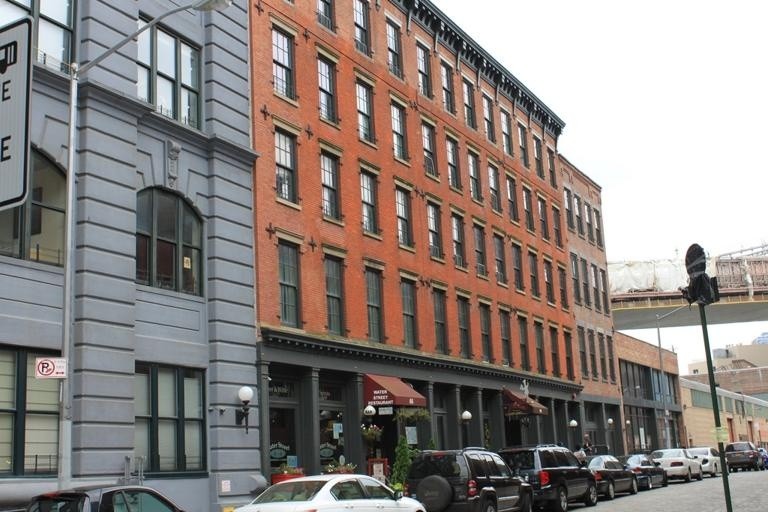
[655,300,701,448]
[55,0,235,487]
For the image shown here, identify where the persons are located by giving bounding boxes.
[574,444,586,464]
[583,433,592,449]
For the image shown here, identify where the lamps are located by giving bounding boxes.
[623,419,632,432]
[232,385,255,435]
[566,417,578,432]
[605,416,615,431]
[457,407,473,428]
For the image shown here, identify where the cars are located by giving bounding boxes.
[233,474,425,511]
[319,409,336,420]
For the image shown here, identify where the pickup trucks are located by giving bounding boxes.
[27,486,186,512]
